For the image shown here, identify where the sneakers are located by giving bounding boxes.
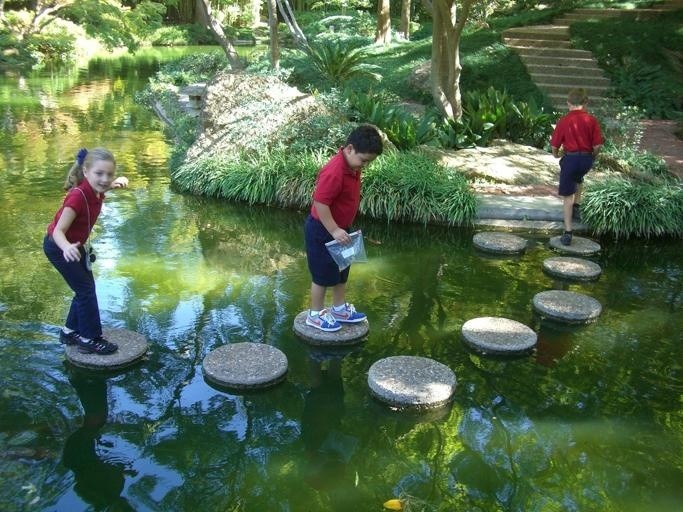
[58,328,80,345]
[561,232,572,246]
[305,308,342,332]
[78,336,118,354]
[572,204,579,217]
[331,303,367,323]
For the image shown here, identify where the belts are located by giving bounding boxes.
[567,152,593,156]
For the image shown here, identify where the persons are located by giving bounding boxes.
[304,126,383,331]
[551,88,603,245]
[43,148,128,354]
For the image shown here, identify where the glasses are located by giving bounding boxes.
[89,246,95,262]
